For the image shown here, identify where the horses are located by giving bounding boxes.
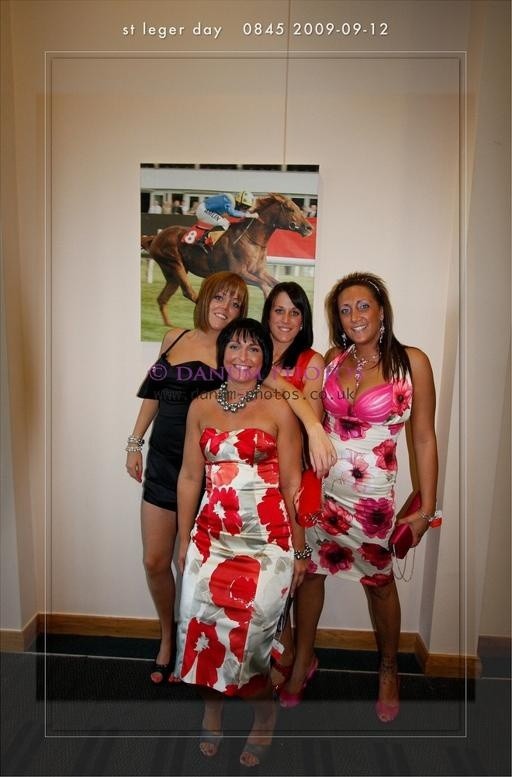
[141,191,314,326]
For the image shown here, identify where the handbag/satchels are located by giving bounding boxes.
[389,489,432,582]
[295,470,323,529]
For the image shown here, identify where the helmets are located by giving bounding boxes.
[234,191,256,208]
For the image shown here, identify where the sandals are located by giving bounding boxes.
[269,645,296,694]
[197,705,223,759]
[238,712,275,771]
[147,638,174,687]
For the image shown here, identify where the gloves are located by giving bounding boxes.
[245,211,260,219]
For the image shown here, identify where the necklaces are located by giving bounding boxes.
[215,376,264,414]
[353,347,390,391]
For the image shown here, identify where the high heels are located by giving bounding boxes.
[278,653,318,710]
[376,671,401,724]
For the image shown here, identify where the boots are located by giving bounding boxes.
[196,225,225,254]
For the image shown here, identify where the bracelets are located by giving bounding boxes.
[128,433,147,444]
[418,508,434,520]
[125,445,145,451]
[292,542,314,560]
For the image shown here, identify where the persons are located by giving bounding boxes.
[299,203,317,217]
[262,280,331,712]
[196,190,262,255]
[146,198,199,216]
[122,270,339,692]
[276,271,438,726]
[172,315,315,766]
